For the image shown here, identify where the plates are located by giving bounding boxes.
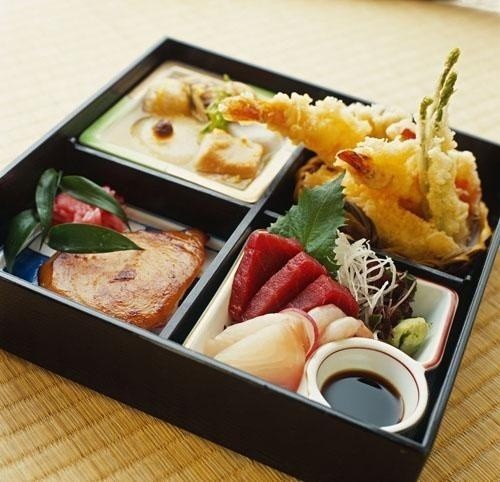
[180,225,460,435]
[82,60,308,205]
[1,189,224,333]
